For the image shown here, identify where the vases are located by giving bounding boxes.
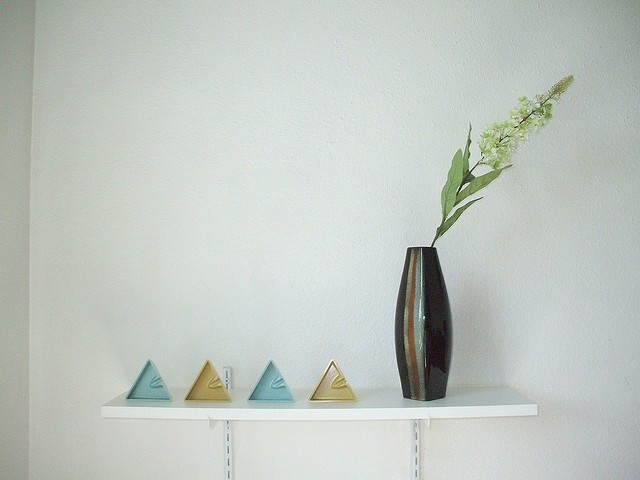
[395,247,452,401]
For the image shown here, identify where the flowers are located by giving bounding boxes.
[430,75,575,248]
